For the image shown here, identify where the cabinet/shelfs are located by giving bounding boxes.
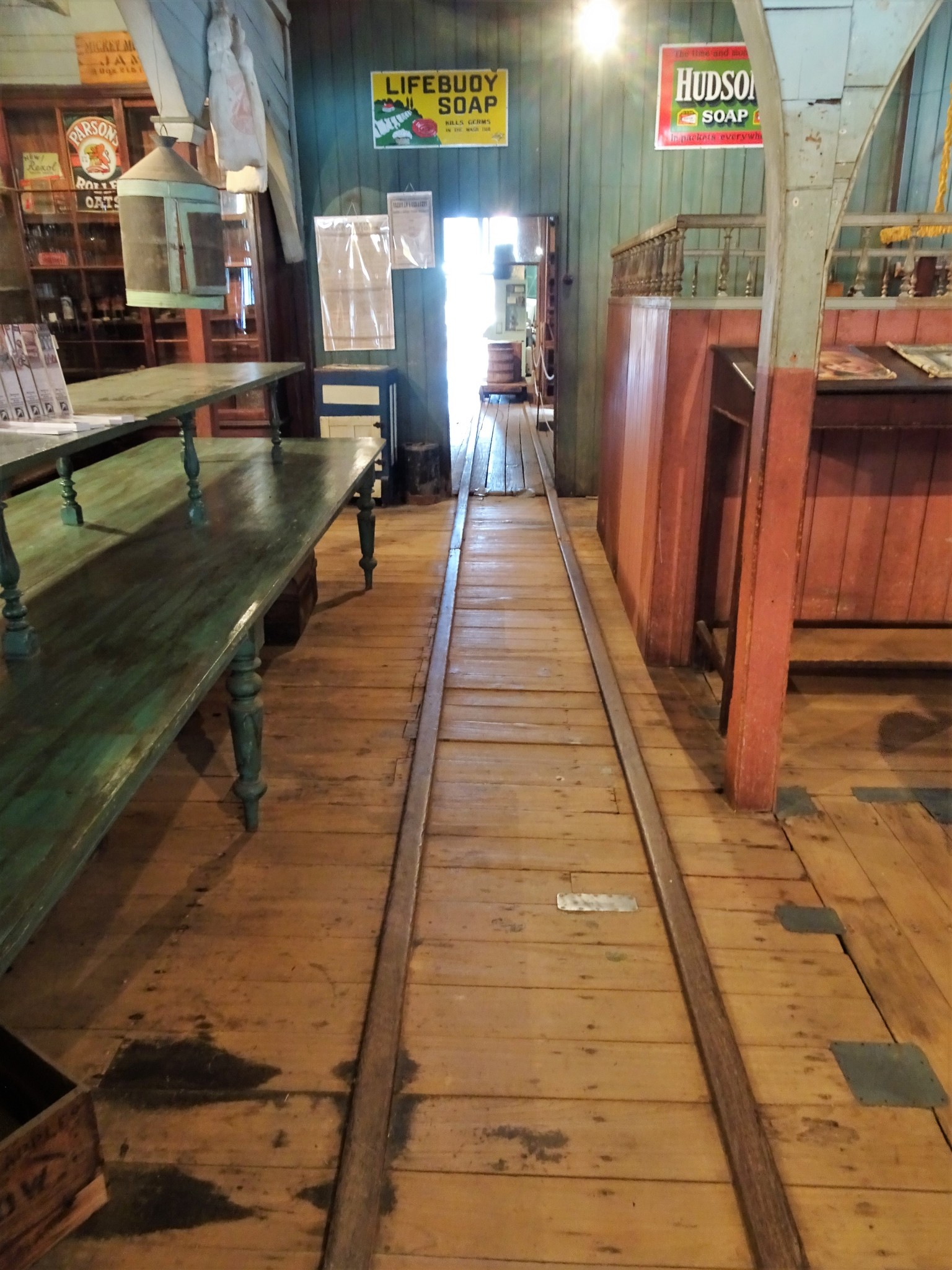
[0,84,271,437]
[314,363,399,507]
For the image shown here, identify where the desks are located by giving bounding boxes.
[0,361,385,977]
[690,344,952,738]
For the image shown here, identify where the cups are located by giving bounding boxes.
[914,256,937,295]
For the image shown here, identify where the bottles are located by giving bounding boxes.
[94,280,127,319]
[60,273,75,321]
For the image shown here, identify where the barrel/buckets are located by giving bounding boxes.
[486,342,514,384]
